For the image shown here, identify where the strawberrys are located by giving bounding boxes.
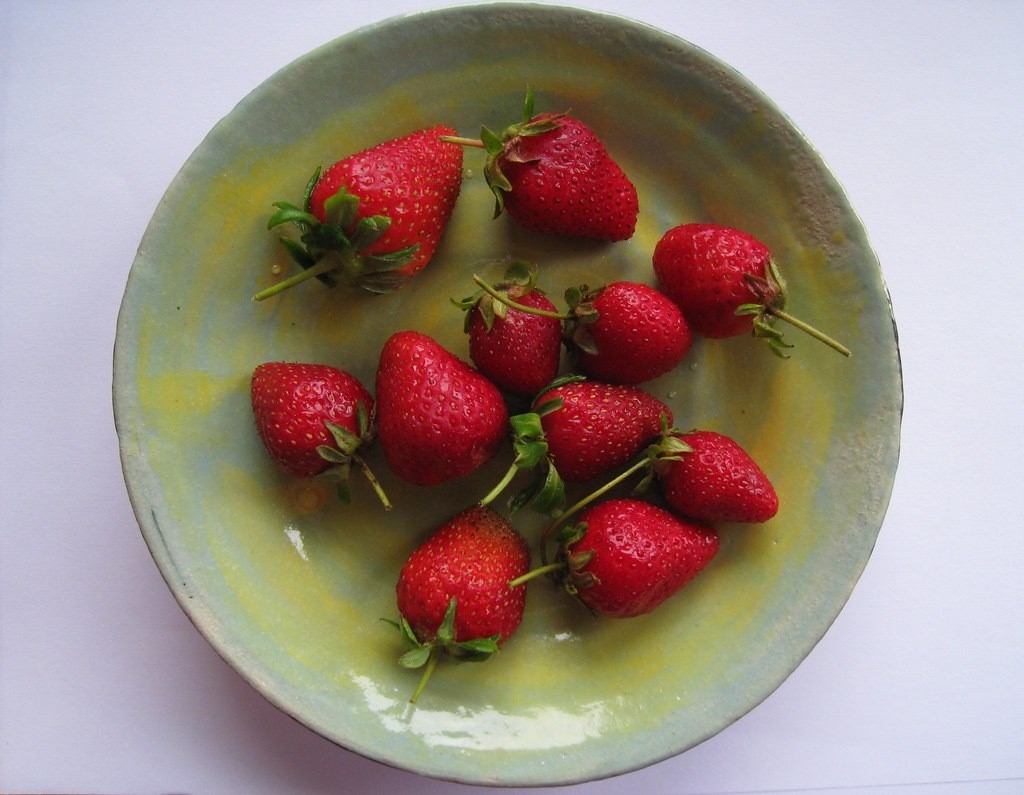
[251,85,787,663]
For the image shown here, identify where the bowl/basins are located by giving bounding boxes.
[109,1,905,787]
